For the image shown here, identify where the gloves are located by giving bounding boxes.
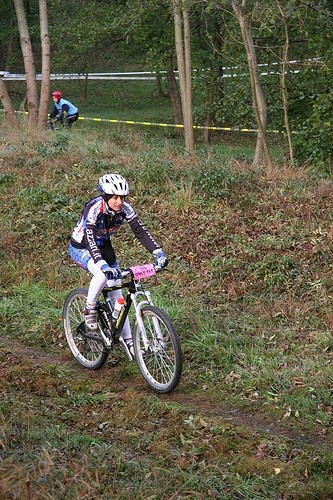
[102,267,122,281]
[155,256,169,268]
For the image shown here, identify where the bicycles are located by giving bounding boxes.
[62,259,183,395]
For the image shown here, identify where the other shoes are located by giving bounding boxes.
[127,340,145,357]
[81,304,98,330]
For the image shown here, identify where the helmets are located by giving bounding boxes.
[97,174,130,197]
[52,91,62,97]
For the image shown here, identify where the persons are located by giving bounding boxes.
[68,174,168,357]
[48,91,79,131]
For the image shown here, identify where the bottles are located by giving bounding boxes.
[111,298,124,319]
